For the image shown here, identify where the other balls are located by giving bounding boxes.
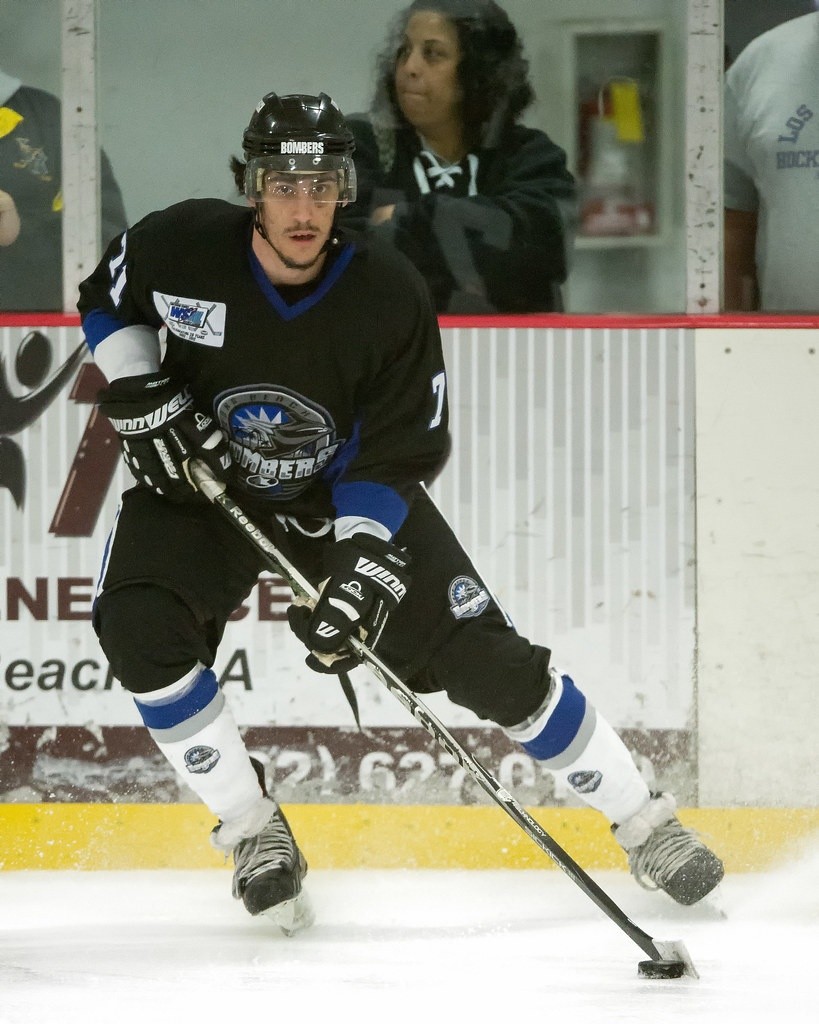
[637,959,684,980]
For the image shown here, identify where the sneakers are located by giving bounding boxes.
[610,790,730,919]
[210,756,315,937]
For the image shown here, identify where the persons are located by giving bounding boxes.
[0,72,128,312]
[724,11,819,314]
[78,93,725,936]
[341,0,576,314]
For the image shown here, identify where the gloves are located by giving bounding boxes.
[287,533,413,674]
[96,368,231,505]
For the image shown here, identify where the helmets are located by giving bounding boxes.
[242,91,357,203]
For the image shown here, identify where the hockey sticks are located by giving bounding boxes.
[189,461,702,983]
